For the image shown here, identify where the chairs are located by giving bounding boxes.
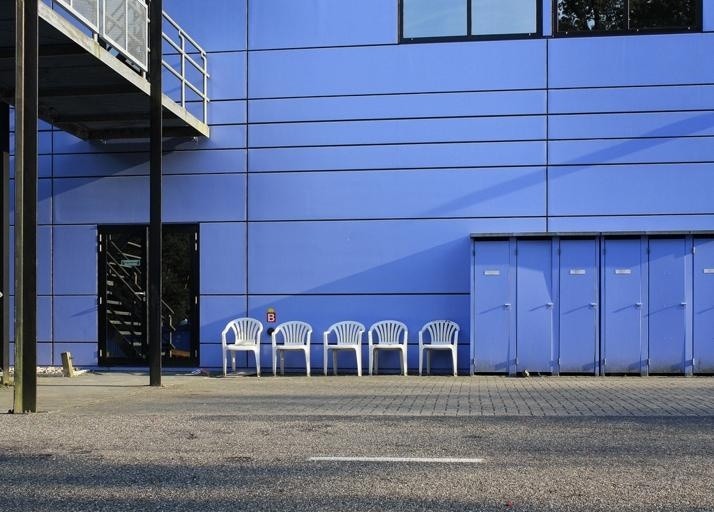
[221,317,462,378]
